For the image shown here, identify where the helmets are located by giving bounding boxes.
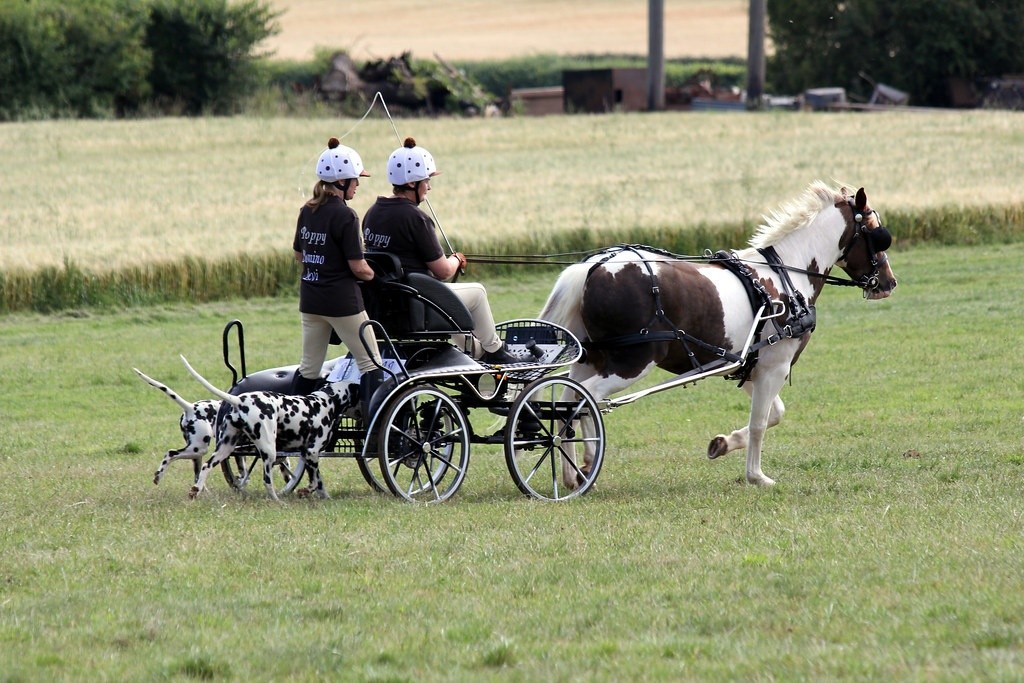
[315,137,371,183]
[388,137,442,185]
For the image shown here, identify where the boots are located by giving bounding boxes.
[359,368,387,429]
[289,369,323,398]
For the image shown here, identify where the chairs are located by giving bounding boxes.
[354,248,475,335]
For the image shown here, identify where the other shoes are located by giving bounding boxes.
[480,344,518,364]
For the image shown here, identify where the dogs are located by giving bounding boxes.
[182,355,365,510]
[132,366,300,498]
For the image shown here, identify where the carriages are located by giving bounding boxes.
[215,176,898,507]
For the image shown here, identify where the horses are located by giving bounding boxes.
[530,180,899,487]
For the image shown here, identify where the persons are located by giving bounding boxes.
[286,135,406,431]
[359,136,535,363]
